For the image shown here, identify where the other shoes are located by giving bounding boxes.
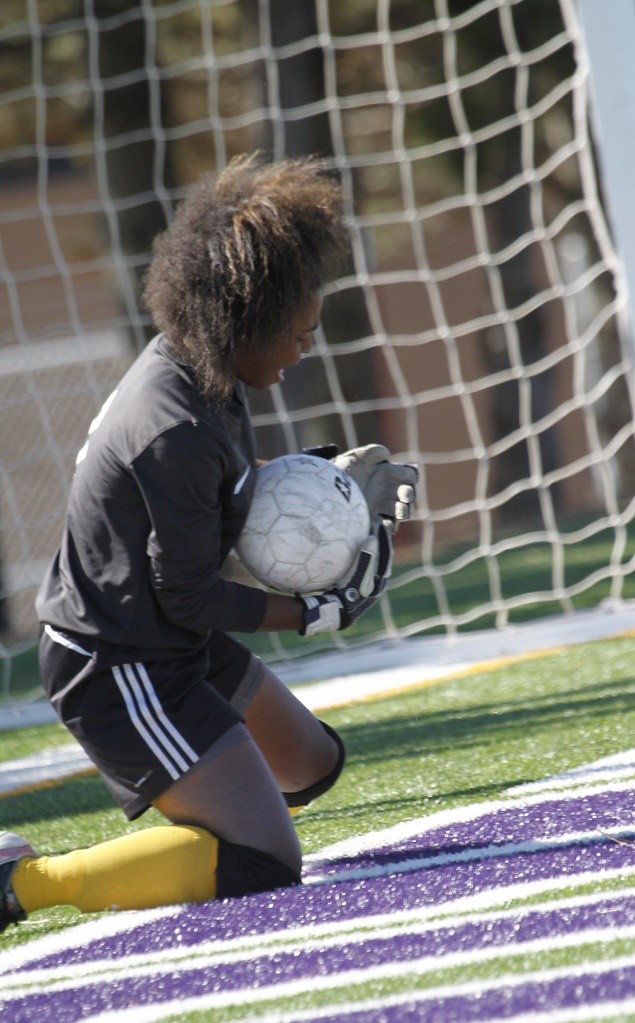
[0,830,39,934]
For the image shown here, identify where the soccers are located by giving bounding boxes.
[235,454,370,595]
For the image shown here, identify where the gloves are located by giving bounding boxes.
[297,523,395,638]
[301,443,419,535]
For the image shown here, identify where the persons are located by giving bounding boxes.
[0,150,419,933]
